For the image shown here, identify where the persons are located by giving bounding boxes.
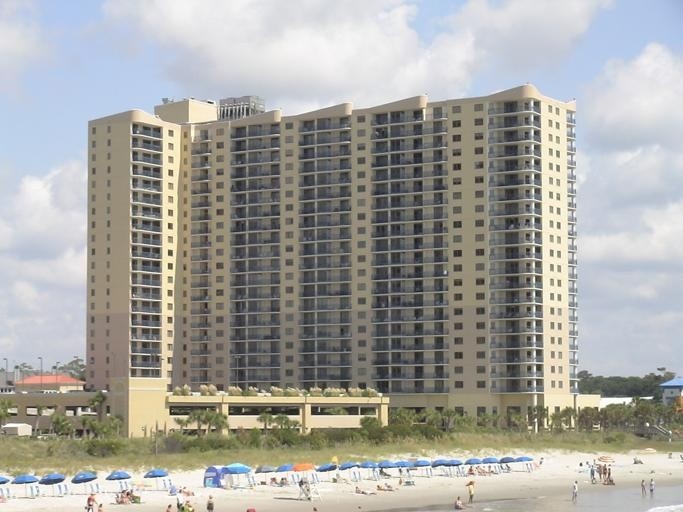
[455,496,465,509]
[580,459,613,483]
[649,478,654,495]
[466,479,475,503]
[572,480,578,502]
[86,487,216,512]
[641,479,647,496]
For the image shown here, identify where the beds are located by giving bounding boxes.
[0,476,10,485]
[11,475,38,498]
[144,469,168,490]
[106,469,131,492]
[71,470,97,492]
[39,474,65,497]
